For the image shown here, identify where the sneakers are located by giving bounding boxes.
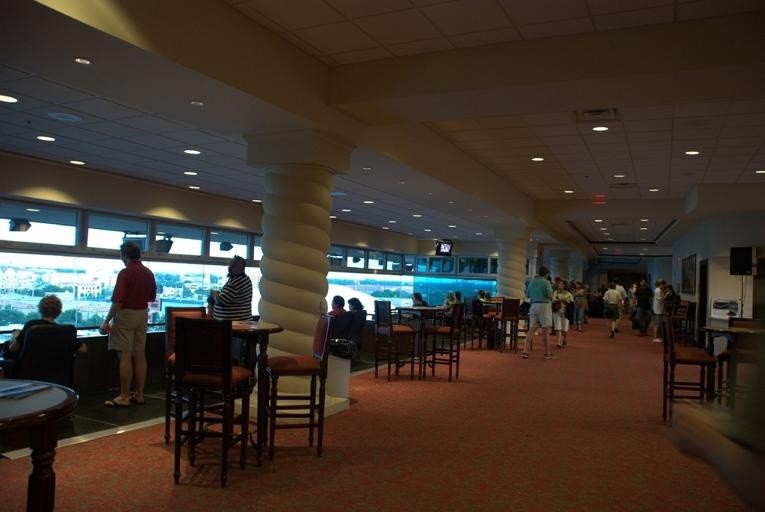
[544,354,557,359]
[520,352,529,357]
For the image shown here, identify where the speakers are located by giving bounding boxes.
[730,246,752,275]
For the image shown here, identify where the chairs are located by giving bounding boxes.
[672,301,689,336]
[717,317,762,405]
[661,315,716,426]
[6,324,77,384]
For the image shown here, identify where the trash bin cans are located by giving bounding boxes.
[325,339,355,397]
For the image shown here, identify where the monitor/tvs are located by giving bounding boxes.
[434,241,454,257]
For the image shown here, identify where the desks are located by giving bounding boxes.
[0,378,79,512]
[703,326,764,411]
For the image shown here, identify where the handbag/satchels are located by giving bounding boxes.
[552,301,561,312]
[519,301,530,316]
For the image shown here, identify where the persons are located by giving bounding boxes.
[443,292,464,328]
[328,296,346,315]
[472,290,499,350]
[348,297,363,311]
[412,292,428,306]
[520,266,560,360]
[553,277,676,347]
[100,242,157,406]
[209,254,253,321]
[3,295,88,365]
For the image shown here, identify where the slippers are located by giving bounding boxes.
[129,395,145,405]
[104,399,130,408]
